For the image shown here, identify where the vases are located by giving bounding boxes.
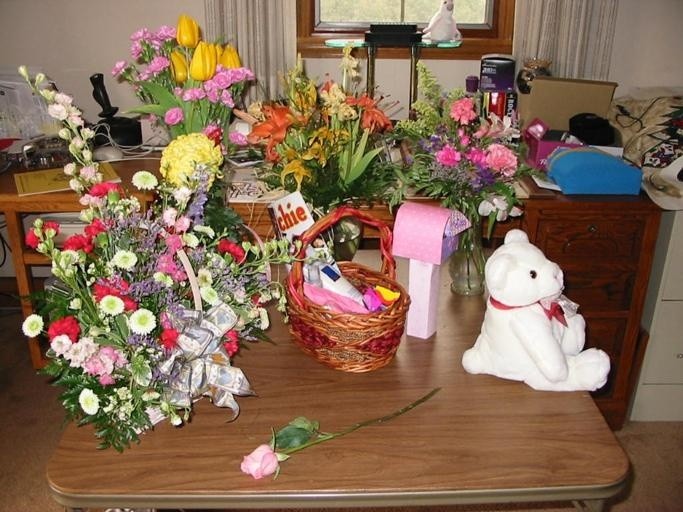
[447,213,488,297]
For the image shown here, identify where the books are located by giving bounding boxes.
[226,147,288,204]
[14,160,122,198]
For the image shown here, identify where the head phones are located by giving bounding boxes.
[517,68,534,95]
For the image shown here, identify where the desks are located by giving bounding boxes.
[44,249,633,512]
[0,146,404,367]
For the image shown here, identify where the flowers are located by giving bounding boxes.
[243,41,398,221]
[396,60,526,286]
[15,67,344,459]
[236,386,445,479]
[112,12,254,152]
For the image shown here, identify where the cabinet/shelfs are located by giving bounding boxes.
[529,150,661,431]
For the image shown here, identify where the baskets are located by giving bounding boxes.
[127,216,207,434]
[282,205,411,374]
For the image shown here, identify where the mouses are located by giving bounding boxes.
[92,147,124,160]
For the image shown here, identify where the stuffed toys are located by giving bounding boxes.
[422,0,462,43]
[463,230,612,392]
